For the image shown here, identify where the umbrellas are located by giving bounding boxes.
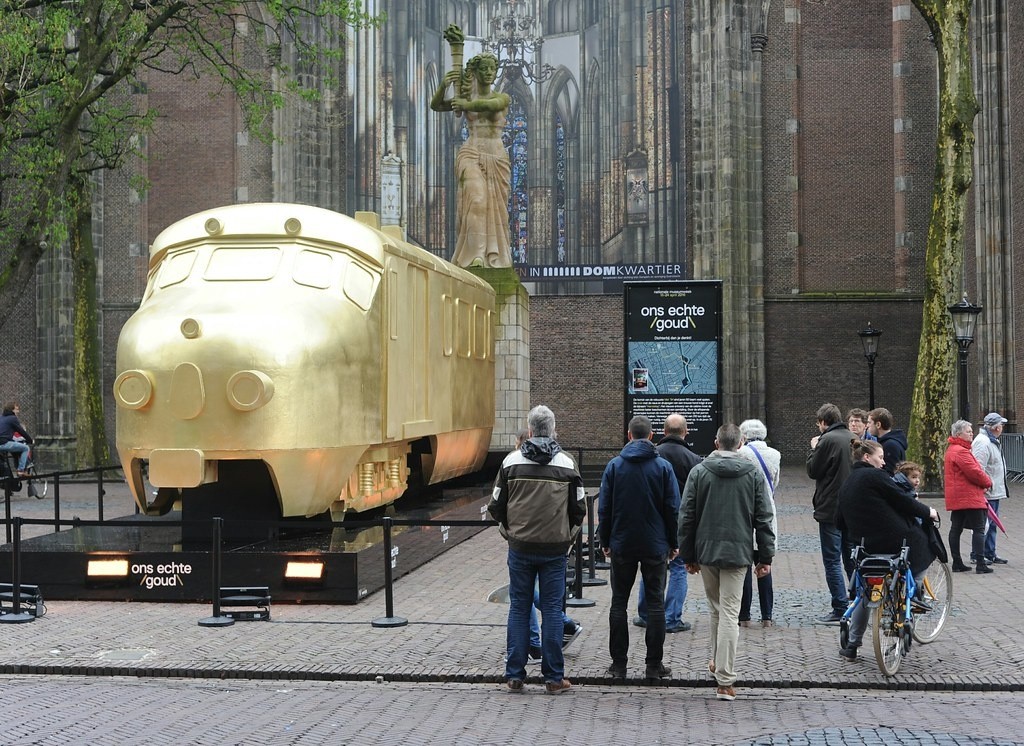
[983,496,1009,539]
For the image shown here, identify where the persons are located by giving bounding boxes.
[430,52,512,268]
[0,401,33,476]
[515,430,582,664]
[737,419,781,627]
[891,461,922,524]
[970,413,1009,564]
[848,408,877,442]
[944,420,993,574]
[806,403,856,625]
[632,413,702,631]
[677,424,776,700]
[596,416,680,676]
[488,405,587,696]
[834,439,938,661]
[867,408,908,477]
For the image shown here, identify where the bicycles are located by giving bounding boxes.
[0,438,48,502]
[851,512,954,678]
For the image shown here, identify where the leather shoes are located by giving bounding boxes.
[716,685,736,700]
[708,660,716,678]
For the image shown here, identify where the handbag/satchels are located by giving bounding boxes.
[922,521,948,563]
[951,502,988,530]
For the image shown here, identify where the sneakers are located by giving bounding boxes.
[561,620,583,651]
[986,555,1008,564]
[507,677,524,692]
[970,557,993,565]
[546,678,571,694]
[504,651,543,665]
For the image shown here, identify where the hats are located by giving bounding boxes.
[984,413,1008,426]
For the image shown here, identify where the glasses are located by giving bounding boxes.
[816,421,821,426]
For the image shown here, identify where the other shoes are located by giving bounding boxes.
[646,663,672,679]
[666,621,691,632]
[739,621,750,627]
[633,616,647,627]
[813,612,848,626]
[976,559,994,573]
[16,471,32,477]
[847,595,855,605]
[762,620,771,628]
[910,580,932,611]
[607,663,626,677]
[952,557,972,571]
[839,644,857,662]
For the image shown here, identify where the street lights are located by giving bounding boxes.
[856,321,883,412]
[946,292,983,423]
